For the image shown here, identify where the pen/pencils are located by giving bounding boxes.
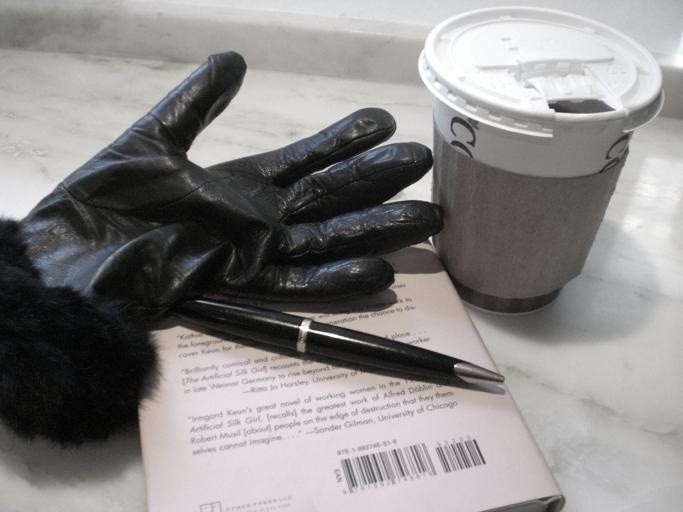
[169,297,507,389]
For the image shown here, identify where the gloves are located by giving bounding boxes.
[0,52,445,444]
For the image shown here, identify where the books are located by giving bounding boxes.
[134,228,567,512]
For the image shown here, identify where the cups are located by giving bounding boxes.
[417,5,666,319]
